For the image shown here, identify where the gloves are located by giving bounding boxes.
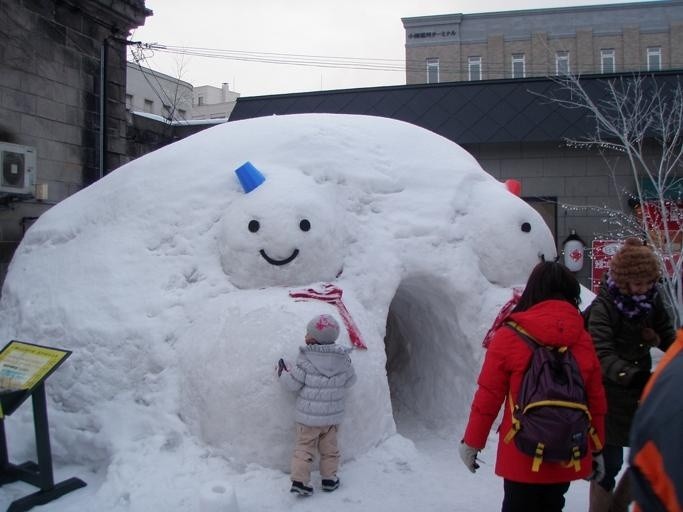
[584,451,604,485]
[459,440,480,474]
[276,358,291,376]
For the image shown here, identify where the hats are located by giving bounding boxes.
[610,237,660,293]
[307,313,340,344]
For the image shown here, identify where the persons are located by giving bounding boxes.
[276,314,357,496]
[458,260,609,512]
[581,237,675,512]
[624,328,683,512]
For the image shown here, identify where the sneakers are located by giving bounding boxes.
[322,476,340,491]
[290,480,313,496]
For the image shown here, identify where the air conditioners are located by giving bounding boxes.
[0,141,37,196]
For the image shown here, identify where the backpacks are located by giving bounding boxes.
[502,318,590,464]
